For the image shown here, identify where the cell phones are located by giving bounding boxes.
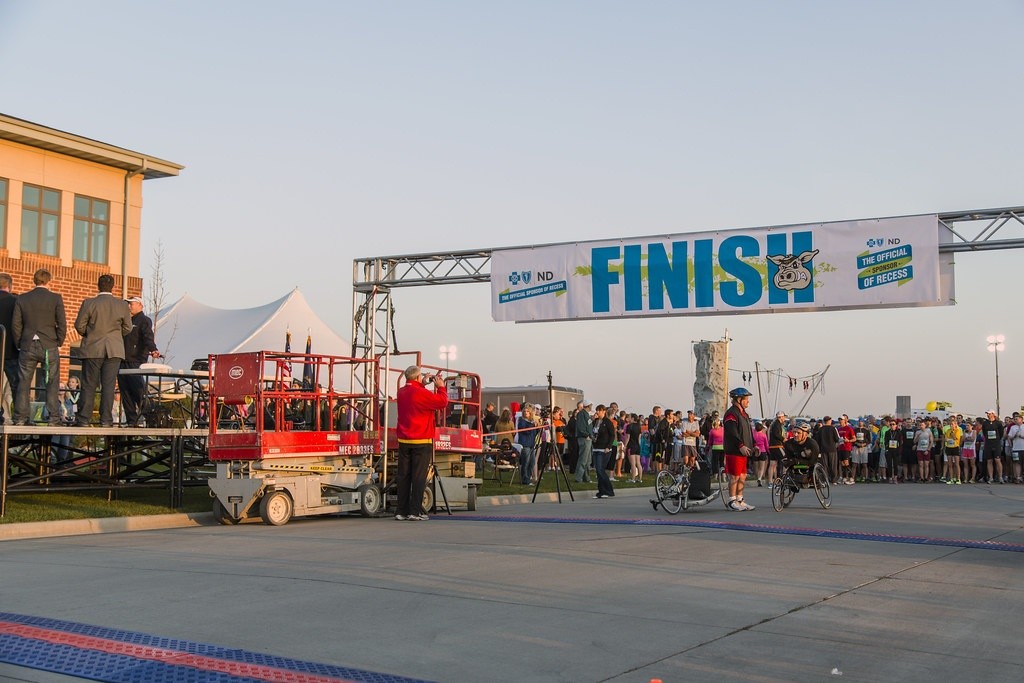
[429,376,435,382]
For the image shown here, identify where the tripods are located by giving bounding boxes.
[531,371,574,504]
[427,440,452,516]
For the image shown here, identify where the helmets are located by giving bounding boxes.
[729,387,752,398]
[793,421,811,434]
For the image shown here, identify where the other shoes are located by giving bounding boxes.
[494,463,671,499]
[756,478,763,487]
[9,417,148,433]
[393,514,407,520]
[767,482,777,489]
[406,514,429,521]
[828,474,1024,487]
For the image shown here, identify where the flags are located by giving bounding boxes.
[303,335,315,423]
[282,331,292,409]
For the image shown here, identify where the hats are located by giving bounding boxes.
[533,403,542,411]
[775,411,788,419]
[122,297,144,307]
[838,416,845,420]
[859,417,865,422]
[985,409,997,416]
[582,400,593,406]
[501,437,512,448]
[687,410,696,415]
[293,377,303,386]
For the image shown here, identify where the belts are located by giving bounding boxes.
[31,340,40,343]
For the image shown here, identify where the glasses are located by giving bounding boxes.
[889,423,896,426]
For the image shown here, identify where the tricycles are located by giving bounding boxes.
[649,455,737,514]
[772,456,832,513]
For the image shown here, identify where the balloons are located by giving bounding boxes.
[926,401,936,413]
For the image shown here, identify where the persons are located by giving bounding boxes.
[0,272,38,426]
[41,376,82,477]
[197,380,250,421]
[723,388,761,510]
[471,403,727,485]
[74,274,132,428]
[393,366,448,521]
[264,377,359,428]
[753,411,1024,490]
[111,386,130,467]
[592,404,616,500]
[115,295,160,429]
[12,268,66,425]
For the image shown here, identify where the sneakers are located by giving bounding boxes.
[724,496,747,511]
[736,495,756,511]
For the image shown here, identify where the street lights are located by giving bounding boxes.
[985,334,1004,418]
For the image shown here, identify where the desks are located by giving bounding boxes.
[118,368,293,431]
[483,446,524,489]
